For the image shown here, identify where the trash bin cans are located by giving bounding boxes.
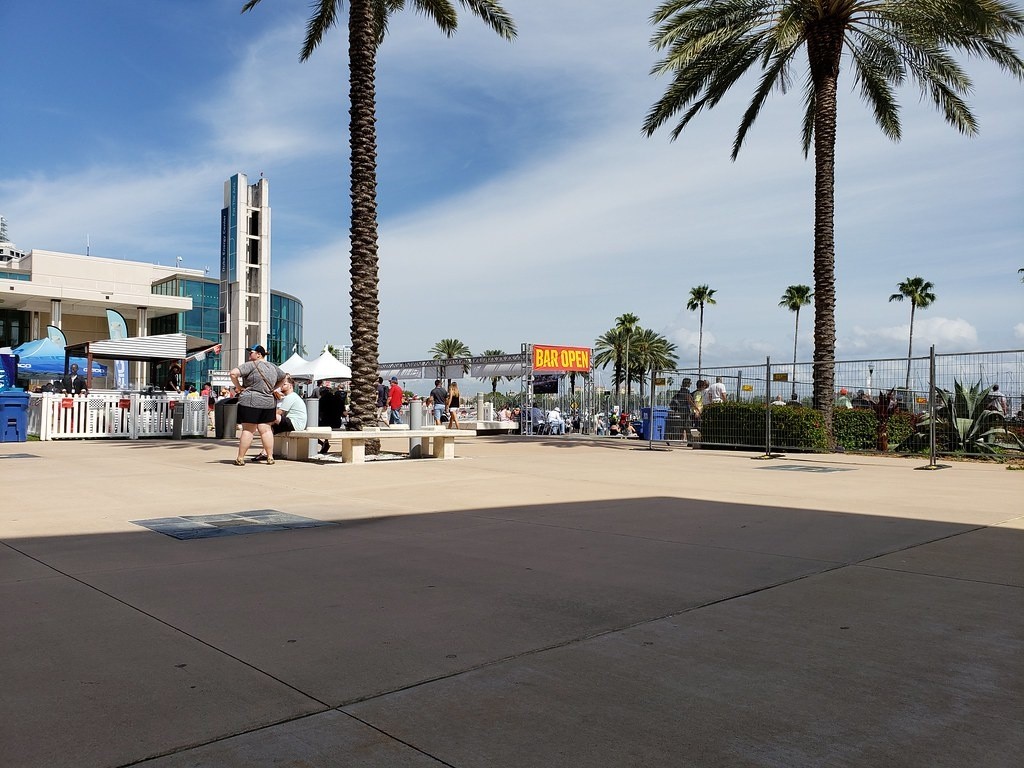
[641,407,668,440]
[215,397,240,439]
[0,391,31,442]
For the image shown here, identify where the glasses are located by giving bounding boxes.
[249,352,257,355]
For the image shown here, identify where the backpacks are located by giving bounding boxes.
[208,391,216,404]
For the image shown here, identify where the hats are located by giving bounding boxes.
[389,377,397,381]
[554,407,560,412]
[840,388,848,395]
[204,382,212,387]
[246,345,266,358]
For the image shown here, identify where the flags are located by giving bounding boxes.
[106,308,130,389]
[47,325,67,345]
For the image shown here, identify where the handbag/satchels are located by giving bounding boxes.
[274,391,281,399]
[440,411,450,422]
[669,392,680,411]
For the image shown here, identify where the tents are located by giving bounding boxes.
[278,352,352,389]
[12,337,107,388]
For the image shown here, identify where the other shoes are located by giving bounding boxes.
[317,442,330,454]
[266,458,275,464]
[209,424,214,431]
[561,432,566,435]
[666,440,670,446]
[251,452,268,464]
[234,459,245,465]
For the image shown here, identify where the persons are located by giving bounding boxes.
[497,404,531,434]
[597,410,635,434]
[427,380,448,425]
[837,389,908,414]
[446,382,460,430]
[532,403,565,434]
[566,414,593,433]
[771,393,802,407]
[188,382,236,431]
[34,364,88,397]
[230,345,287,466]
[989,384,1006,413]
[676,376,726,446]
[167,366,180,393]
[376,376,403,426]
[251,373,349,466]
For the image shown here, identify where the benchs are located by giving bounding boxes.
[255,424,477,463]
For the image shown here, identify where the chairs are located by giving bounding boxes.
[542,417,560,435]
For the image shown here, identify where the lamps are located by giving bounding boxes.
[176,255,183,267]
